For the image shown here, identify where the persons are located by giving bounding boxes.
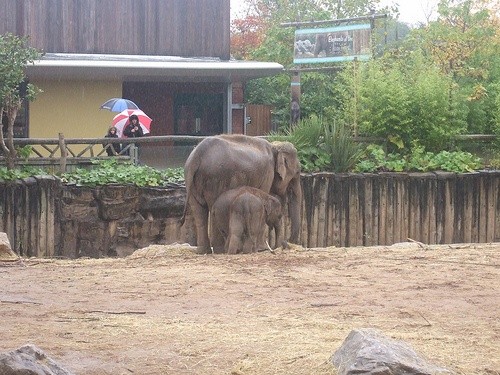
[103,126,121,156]
[123,115,143,155]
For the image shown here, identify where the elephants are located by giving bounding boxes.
[178,134,302,256]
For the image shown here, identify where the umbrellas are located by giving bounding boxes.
[99,98,139,112]
[112,109,153,138]
